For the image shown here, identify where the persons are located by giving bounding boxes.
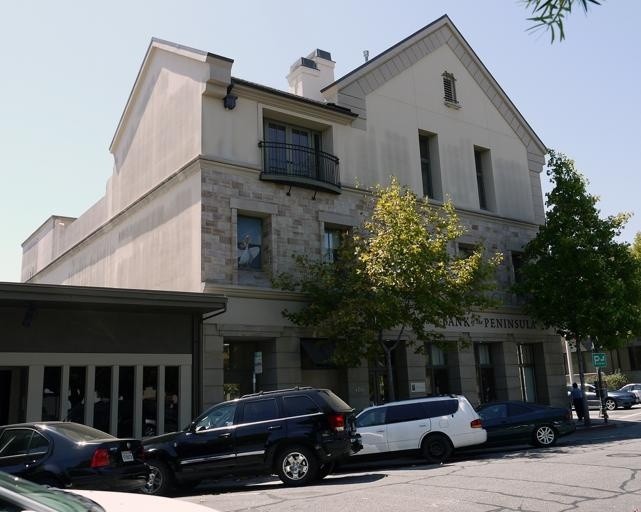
[570,383,585,421]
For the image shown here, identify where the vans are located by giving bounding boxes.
[339,390,488,463]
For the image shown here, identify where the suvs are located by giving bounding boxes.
[142,383,362,498]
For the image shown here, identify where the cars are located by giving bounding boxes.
[137,402,178,439]
[0,468,219,512]
[567,383,638,410]
[0,420,146,490]
[618,383,641,404]
[456,398,576,448]
